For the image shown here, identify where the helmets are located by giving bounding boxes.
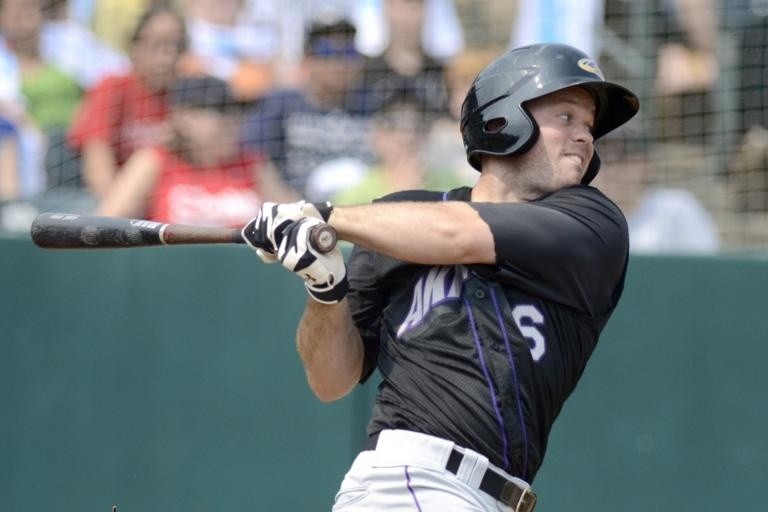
[457,41,642,191]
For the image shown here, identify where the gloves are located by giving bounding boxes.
[269,212,350,304]
[243,197,335,264]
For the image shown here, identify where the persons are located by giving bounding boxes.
[0,0,767,265]
[239,39,642,512]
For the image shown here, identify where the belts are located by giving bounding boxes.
[356,430,541,512]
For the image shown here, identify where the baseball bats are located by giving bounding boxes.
[30,210,335,253]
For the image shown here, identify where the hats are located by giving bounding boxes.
[167,72,258,117]
